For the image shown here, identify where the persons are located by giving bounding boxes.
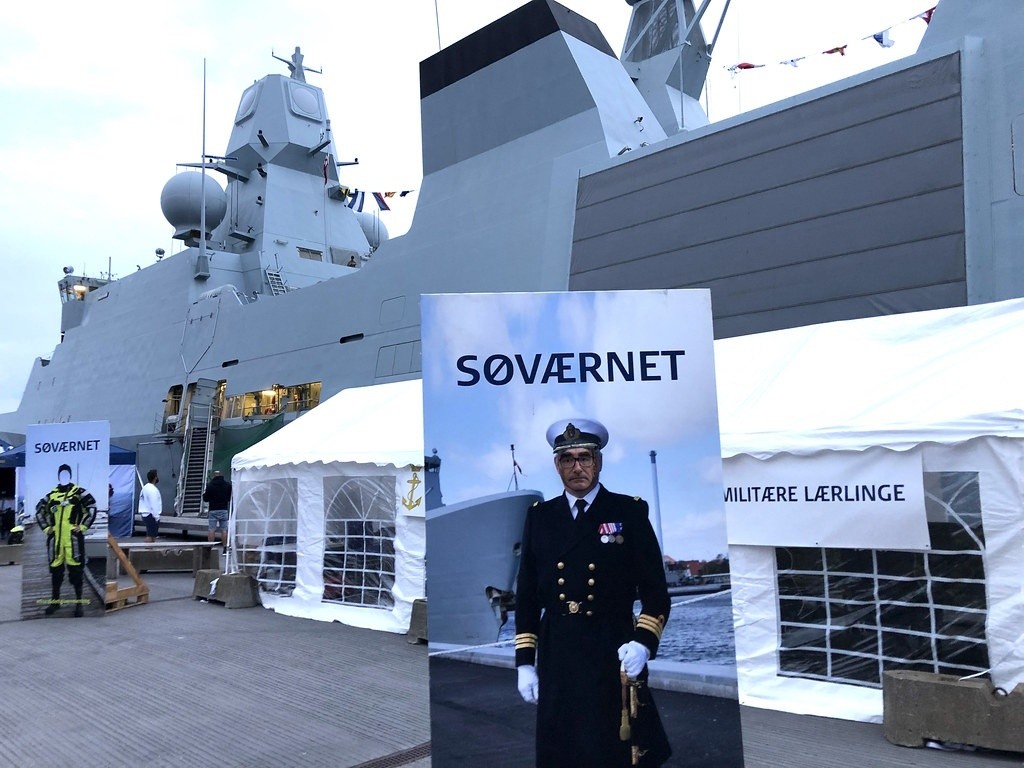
[203,471,231,555]
[35,464,98,616]
[559,448,594,489]
[512,417,673,768]
[138,470,162,542]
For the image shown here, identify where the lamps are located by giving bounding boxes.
[165,413,182,424]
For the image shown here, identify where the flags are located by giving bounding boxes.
[400,190,409,196]
[738,62,762,69]
[374,193,389,211]
[912,7,937,26]
[384,192,395,197]
[782,58,801,67]
[873,30,894,49]
[825,45,847,56]
[348,191,365,212]
[323,153,329,185]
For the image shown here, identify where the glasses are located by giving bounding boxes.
[557,452,597,468]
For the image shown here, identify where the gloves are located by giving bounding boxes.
[618,640,650,678]
[516,666,538,705]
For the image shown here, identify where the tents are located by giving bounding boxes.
[713,297,1024,725]
[0,443,136,537]
[227,379,425,633]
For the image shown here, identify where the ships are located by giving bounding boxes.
[2,0,1024,560]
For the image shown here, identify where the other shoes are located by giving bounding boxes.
[223,547,227,555]
[46,603,59,614]
[75,604,84,618]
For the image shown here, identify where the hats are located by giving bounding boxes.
[58,464,73,481]
[546,417,610,458]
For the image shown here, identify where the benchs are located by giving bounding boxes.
[117,541,223,578]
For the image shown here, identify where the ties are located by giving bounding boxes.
[574,499,589,524]
